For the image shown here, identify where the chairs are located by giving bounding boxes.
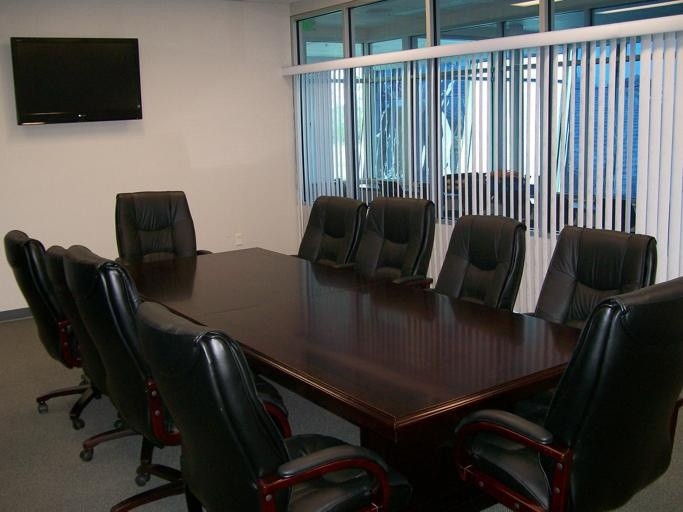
[294,194,366,277]
[439,273,683,511]
[533,225,658,330]
[4,227,181,488]
[435,214,526,312]
[115,191,215,269]
[362,196,435,290]
[110,300,393,511]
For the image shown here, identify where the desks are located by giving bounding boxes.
[114,246,586,512]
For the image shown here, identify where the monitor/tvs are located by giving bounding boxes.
[10,36,142,125]
